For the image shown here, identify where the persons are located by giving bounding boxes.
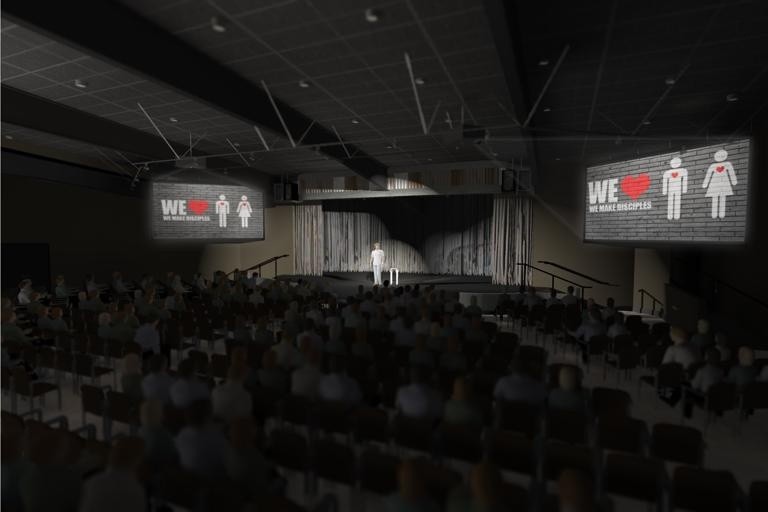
[369,242,385,286]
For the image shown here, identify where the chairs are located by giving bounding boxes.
[1,299,767,512]
